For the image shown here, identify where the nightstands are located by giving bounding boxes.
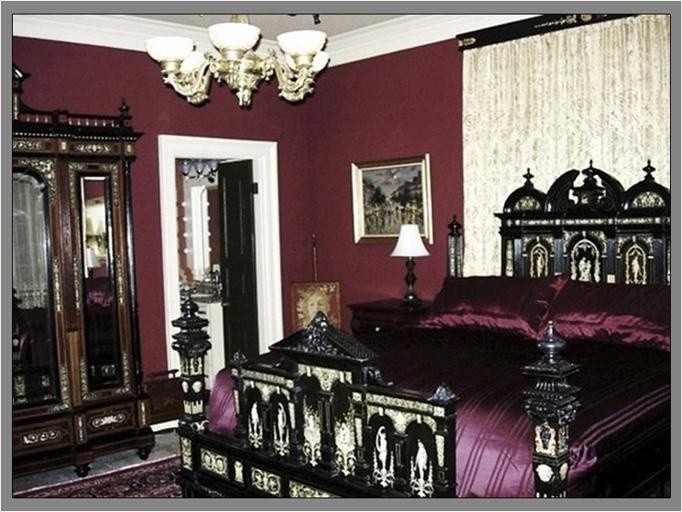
[345,300,433,346]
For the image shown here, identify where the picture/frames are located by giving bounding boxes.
[352,153,435,245]
[290,281,344,334]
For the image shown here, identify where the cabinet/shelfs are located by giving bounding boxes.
[11,65,158,480]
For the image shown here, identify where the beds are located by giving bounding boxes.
[170,155,669,500]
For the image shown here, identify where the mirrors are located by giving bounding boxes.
[65,160,137,403]
[13,159,72,421]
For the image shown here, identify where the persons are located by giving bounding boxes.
[304,289,330,329]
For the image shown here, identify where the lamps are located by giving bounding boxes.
[177,158,223,186]
[147,15,332,108]
[389,222,432,306]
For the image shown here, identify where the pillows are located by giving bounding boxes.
[405,273,671,354]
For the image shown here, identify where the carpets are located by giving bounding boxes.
[12,454,187,498]
